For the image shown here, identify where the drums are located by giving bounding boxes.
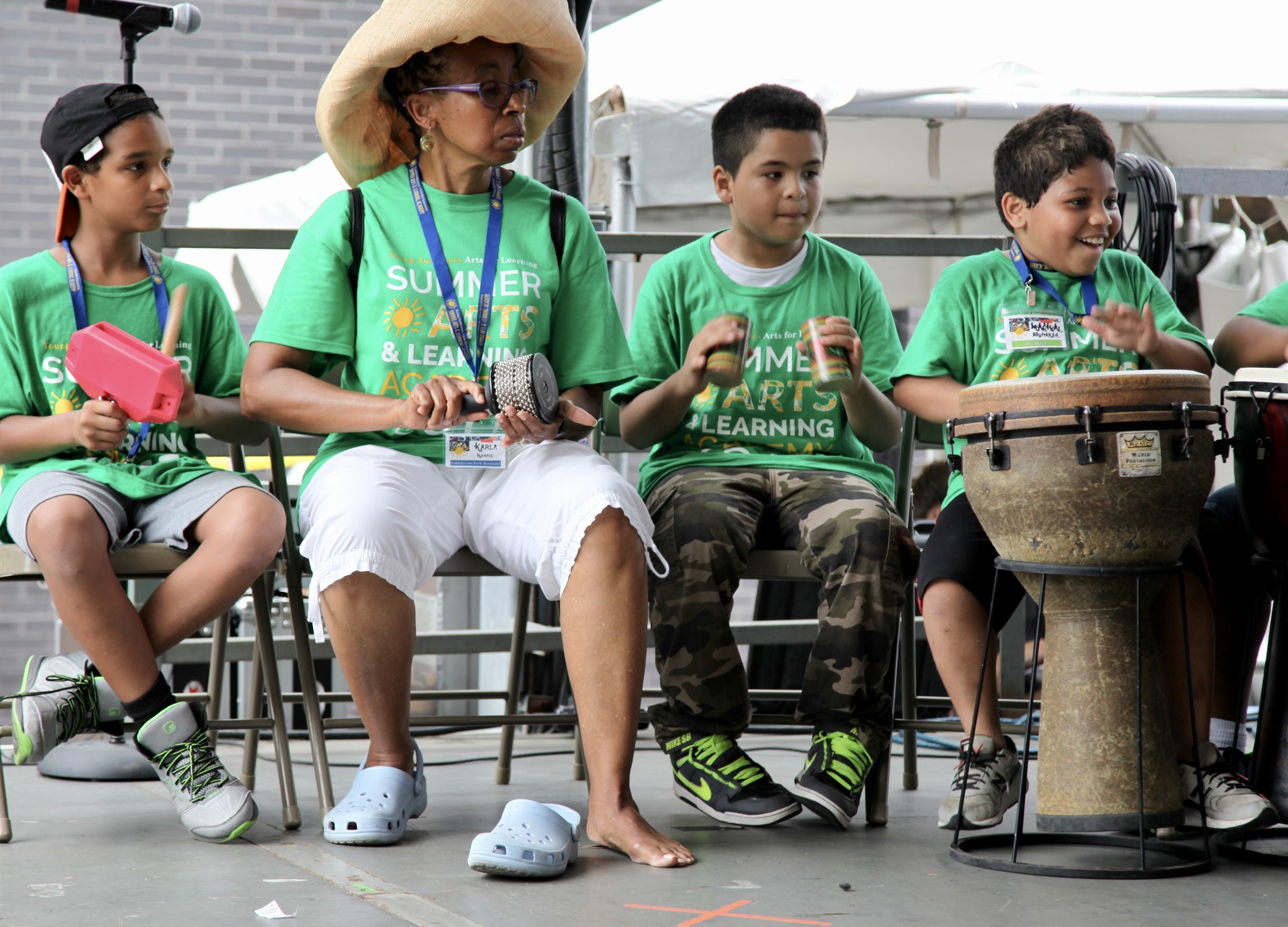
[1220,367,1288,869]
[949,369,1229,881]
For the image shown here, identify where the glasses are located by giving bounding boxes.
[403,79,537,109]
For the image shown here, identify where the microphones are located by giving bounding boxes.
[46,0,203,35]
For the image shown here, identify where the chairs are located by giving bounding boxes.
[0,380,1041,842]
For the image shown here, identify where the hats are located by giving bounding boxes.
[40,84,160,243]
[315,0,586,188]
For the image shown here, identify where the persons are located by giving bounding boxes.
[1213,198,1288,245]
[238,0,696,868]
[609,84,920,829]
[890,106,1276,833]
[0,82,285,842]
[1213,279,1288,811]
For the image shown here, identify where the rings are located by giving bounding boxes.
[514,438,526,446]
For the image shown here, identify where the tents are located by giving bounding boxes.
[173,0,1288,491]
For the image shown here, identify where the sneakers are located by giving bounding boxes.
[795,722,889,831]
[12,651,127,766]
[937,735,1029,830]
[661,733,803,825]
[1185,741,1279,830]
[133,701,258,844]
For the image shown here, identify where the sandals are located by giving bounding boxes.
[323,738,428,843]
[467,799,581,875]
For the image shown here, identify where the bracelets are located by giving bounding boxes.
[554,417,568,439]
